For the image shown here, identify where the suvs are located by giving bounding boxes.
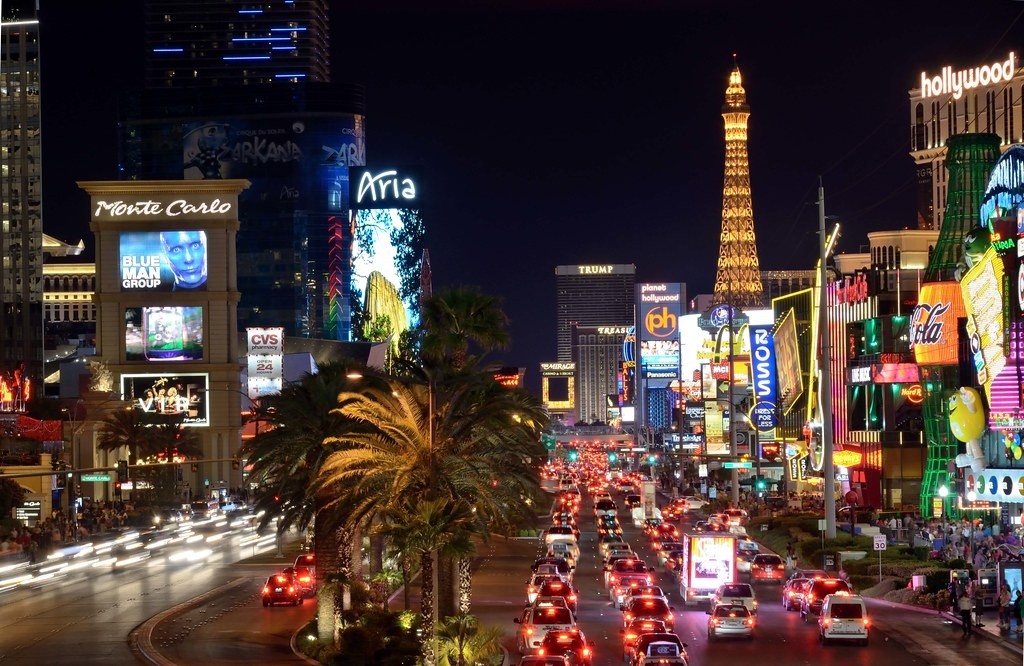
[710,583,758,612]
[750,554,785,584]
[261,573,305,608]
[292,554,317,581]
[800,578,853,624]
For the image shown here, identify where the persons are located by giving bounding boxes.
[639,465,838,518]
[838,489,1024,642]
[0,499,132,564]
[791,548,800,570]
[786,542,793,569]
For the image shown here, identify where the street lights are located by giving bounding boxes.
[61,399,84,537]
[702,392,788,518]
[967,491,976,562]
[197,388,270,416]
[938,485,949,548]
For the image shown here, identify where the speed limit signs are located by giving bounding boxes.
[873,535,886,550]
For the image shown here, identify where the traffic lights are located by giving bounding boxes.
[115,482,120,497]
[569,450,577,460]
[650,449,654,463]
[75,484,80,498]
[756,482,766,490]
[608,448,615,461]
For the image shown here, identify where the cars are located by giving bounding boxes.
[514,446,831,666]
[163,500,257,522]
[817,592,869,643]
[282,567,317,596]
[705,604,755,639]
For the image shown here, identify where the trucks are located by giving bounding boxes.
[676,531,737,606]
